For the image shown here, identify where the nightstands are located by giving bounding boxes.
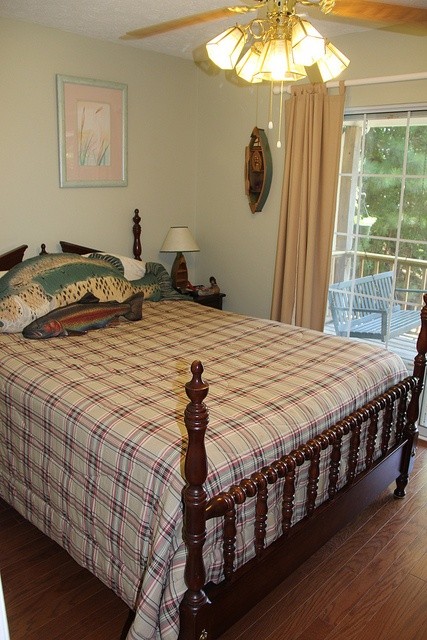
[190,285,226,311]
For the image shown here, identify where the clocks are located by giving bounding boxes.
[244,126,273,215]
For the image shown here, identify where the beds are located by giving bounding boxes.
[0,209,427,639]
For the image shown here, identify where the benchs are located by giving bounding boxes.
[329,271,427,342]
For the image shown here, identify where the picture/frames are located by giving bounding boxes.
[56,73,128,188]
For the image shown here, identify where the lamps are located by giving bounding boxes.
[205,11,352,84]
[159,226,201,292]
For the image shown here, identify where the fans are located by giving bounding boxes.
[126,0,427,40]
